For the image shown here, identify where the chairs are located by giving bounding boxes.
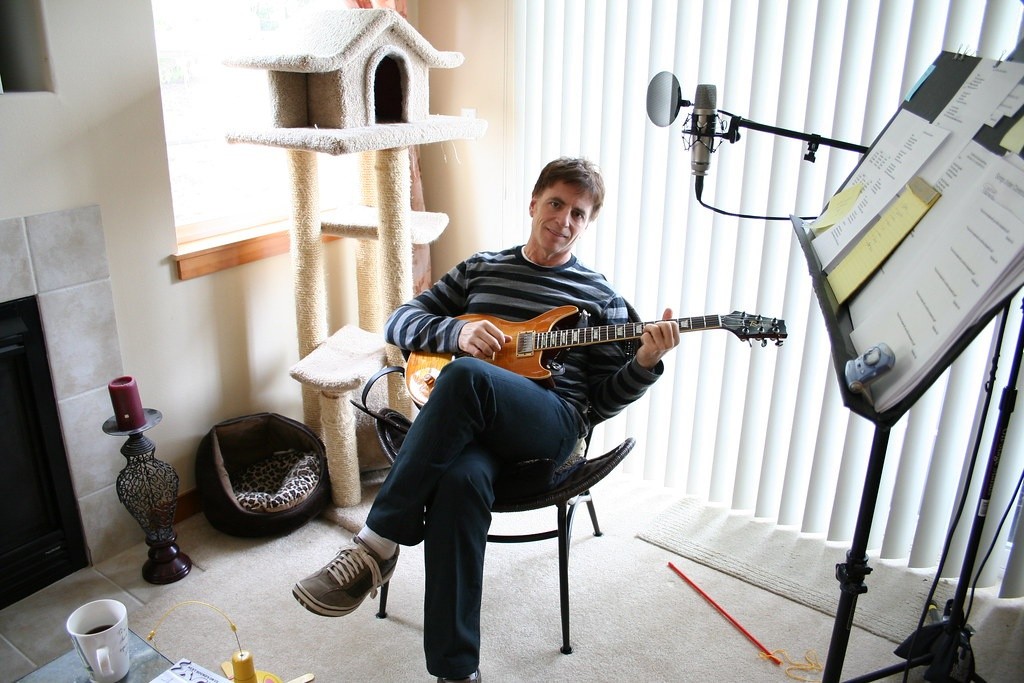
[362,366,636,654]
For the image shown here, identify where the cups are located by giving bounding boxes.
[65,598,131,683]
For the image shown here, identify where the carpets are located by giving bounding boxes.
[127,505,932,683]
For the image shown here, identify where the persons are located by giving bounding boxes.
[291,158,680,683]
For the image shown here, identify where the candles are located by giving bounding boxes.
[108,376,147,431]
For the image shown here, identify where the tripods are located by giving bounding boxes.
[843,311,1024,683]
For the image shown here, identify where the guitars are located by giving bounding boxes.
[402,306,787,410]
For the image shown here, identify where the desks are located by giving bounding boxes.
[14,628,174,683]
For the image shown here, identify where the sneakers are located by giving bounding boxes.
[293,533,399,617]
[437,667,481,683]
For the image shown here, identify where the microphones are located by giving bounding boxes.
[691,84,718,199]
